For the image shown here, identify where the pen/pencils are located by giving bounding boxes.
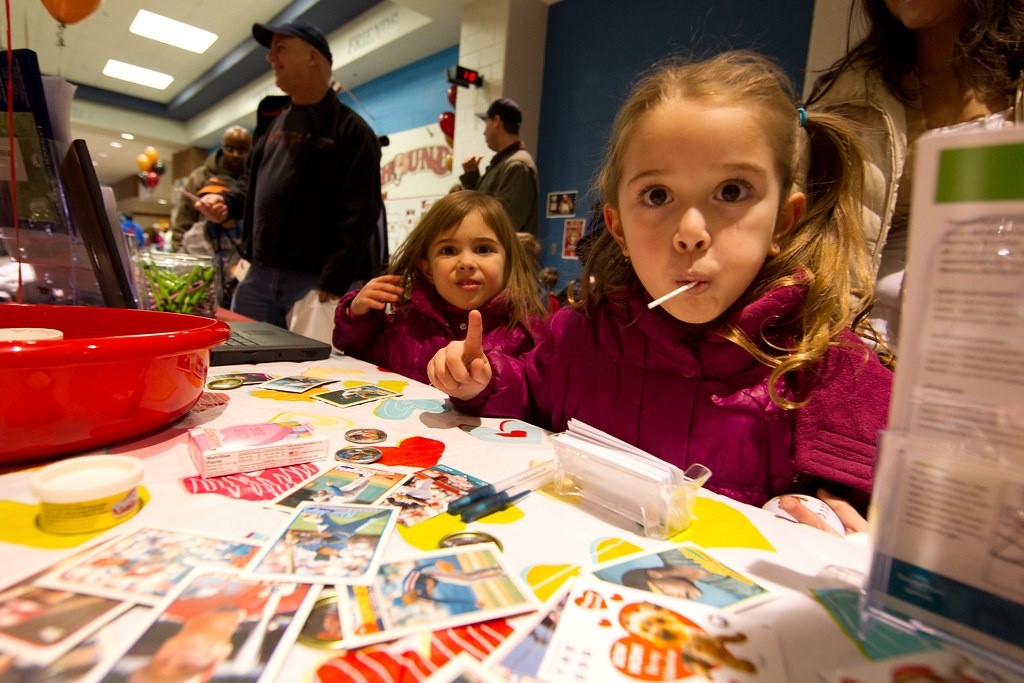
[179,187,200,201]
[460,470,556,524]
[447,460,558,516]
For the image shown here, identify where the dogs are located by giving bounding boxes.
[625,605,756,683]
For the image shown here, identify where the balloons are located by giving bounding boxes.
[136,147,164,188]
[42,0,102,25]
[438,86,457,171]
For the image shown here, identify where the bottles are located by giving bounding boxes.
[136,249,218,313]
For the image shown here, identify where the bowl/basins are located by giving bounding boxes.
[1,300,230,466]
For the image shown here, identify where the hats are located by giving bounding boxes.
[252,17,331,64]
[476,98,521,124]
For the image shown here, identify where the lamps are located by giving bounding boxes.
[444,64,483,89]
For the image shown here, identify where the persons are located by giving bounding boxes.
[120,213,144,246]
[332,190,550,386]
[194,22,384,329]
[558,194,572,211]
[89,475,503,683]
[515,232,559,312]
[146,222,172,246]
[427,48,891,536]
[786,0,1024,361]
[172,125,252,257]
[621,547,763,606]
[342,386,389,399]
[459,98,539,239]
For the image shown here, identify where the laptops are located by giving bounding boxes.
[59,138,331,367]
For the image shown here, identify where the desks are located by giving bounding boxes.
[2,350,866,683]
